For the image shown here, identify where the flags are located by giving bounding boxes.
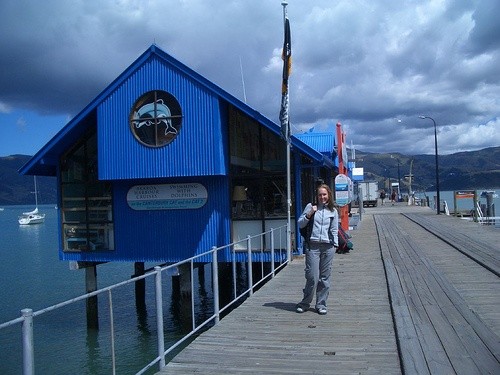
[279,6,293,148]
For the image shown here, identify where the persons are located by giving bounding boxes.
[380,189,386,205]
[391,190,397,205]
[295,184,339,315]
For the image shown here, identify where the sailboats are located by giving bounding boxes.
[22,176,39,217]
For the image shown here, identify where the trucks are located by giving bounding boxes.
[359,182,379,207]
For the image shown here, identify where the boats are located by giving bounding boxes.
[18,214,45,225]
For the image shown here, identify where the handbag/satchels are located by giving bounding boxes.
[299,202,317,243]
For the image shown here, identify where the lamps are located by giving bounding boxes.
[232,186,247,218]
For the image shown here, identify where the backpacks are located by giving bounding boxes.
[335,222,354,254]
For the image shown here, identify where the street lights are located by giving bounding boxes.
[419,115,441,215]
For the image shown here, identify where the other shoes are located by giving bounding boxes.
[317,308,327,314]
[296,307,305,313]
